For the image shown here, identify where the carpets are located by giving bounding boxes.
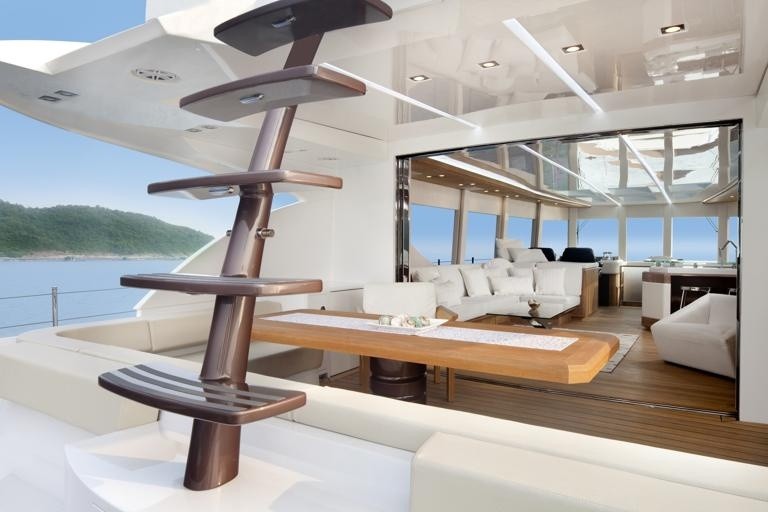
[546,326,639,375]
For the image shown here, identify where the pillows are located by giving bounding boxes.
[496,238,523,262]
[489,277,536,297]
[458,268,492,297]
[437,280,462,308]
[508,247,548,263]
[509,267,534,291]
[534,268,566,297]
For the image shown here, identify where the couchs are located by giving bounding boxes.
[650,293,737,379]
[413,262,598,322]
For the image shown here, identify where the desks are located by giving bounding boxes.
[641,268,737,329]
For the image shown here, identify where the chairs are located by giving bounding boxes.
[679,286,711,310]
[359,282,456,403]
[561,248,596,264]
[432,304,459,384]
[528,247,556,261]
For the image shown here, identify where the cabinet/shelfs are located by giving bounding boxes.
[621,261,654,307]
[600,272,621,306]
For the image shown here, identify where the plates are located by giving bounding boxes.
[367,317,450,334]
